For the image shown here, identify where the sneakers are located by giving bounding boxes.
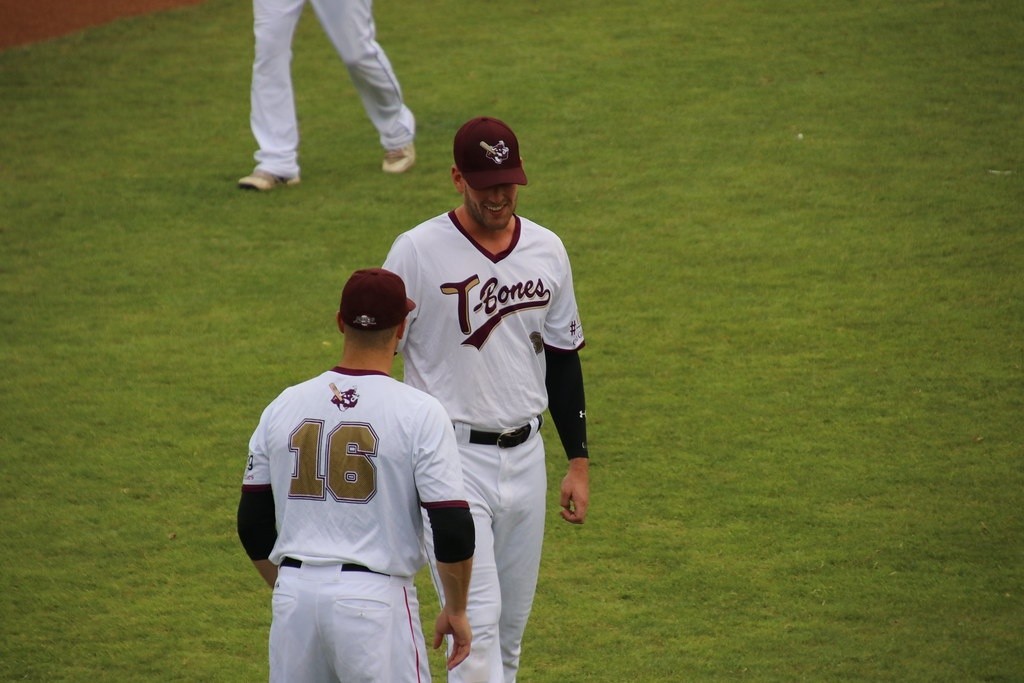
[381,143,415,173]
[238,169,300,191]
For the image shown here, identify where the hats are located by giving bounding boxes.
[454,116,527,191]
[340,267,416,331]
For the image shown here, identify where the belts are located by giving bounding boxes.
[453,414,543,450]
[279,557,377,573]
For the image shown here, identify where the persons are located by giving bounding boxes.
[237,267,477,683]
[380,115,590,683]
[237,0,416,190]
